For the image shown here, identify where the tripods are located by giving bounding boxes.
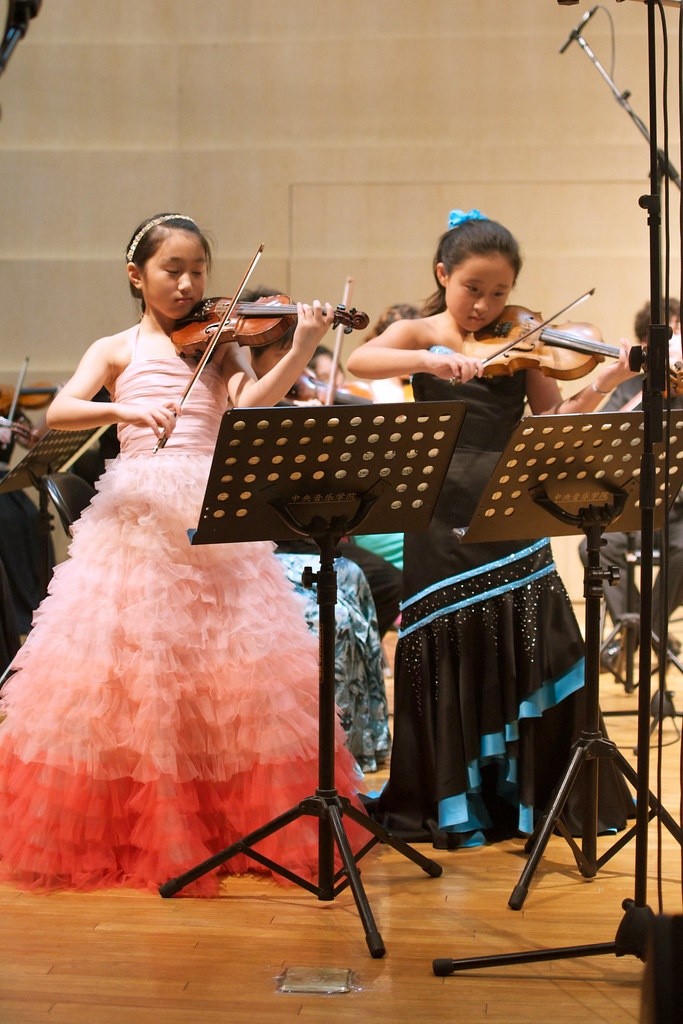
[162,0,683,976]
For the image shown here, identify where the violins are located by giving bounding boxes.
[0,378,59,416]
[171,296,370,359]
[463,305,683,400]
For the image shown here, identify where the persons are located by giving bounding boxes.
[1,215,369,897]
[0,289,683,783]
[347,208,647,851]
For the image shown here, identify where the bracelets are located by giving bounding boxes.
[591,381,617,396]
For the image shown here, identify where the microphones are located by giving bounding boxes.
[0,0,43,72]
[558,5,600,55]
